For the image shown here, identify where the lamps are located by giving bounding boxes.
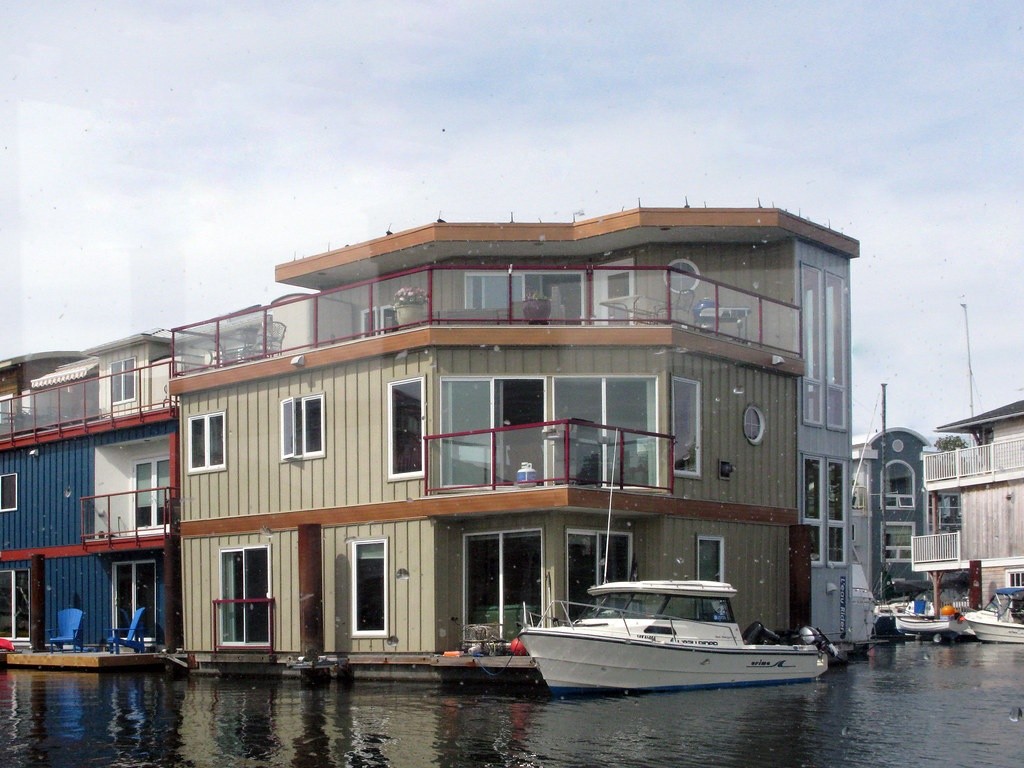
[826,583,837,593]
[29,449,38,457]
[772,355,785,365]
[290,356,304,366]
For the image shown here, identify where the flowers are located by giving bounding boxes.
[392,287,428,311]
[525,285,552,299]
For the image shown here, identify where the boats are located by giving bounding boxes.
[872,585,977,641]
[517,429,848,694]
[961,586,1024,644]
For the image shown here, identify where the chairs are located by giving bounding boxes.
[268,321,287,358]
[45,608,83,653]
[654,289,696,323]
[104,607,146,654]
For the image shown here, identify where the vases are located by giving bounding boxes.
[523,299,551,325]
[396,305,427,330]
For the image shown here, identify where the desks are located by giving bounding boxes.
[599,294,674,325]
[189,326,279,371]
